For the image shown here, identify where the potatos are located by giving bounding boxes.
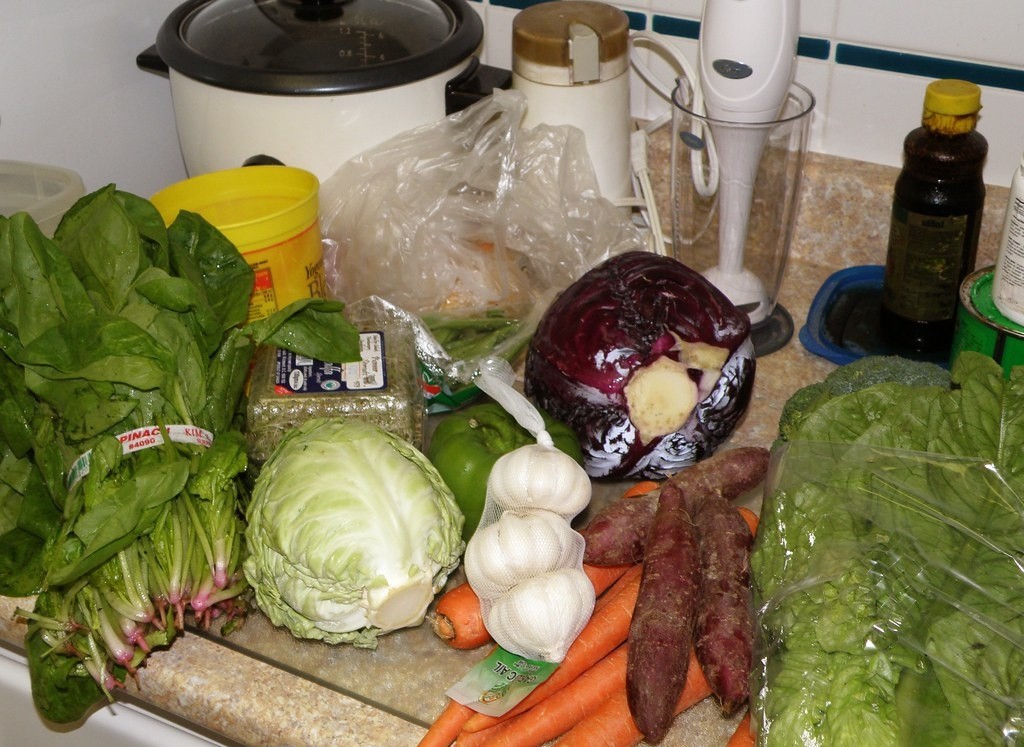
[579,437,769,747]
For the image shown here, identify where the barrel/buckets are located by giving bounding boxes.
[144,166,329,338]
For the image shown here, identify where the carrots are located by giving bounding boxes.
[421,482,714,747]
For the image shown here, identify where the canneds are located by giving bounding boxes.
[952,263,1024,385]
[991,154,1024,327]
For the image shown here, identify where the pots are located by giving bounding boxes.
[136,0,513,188]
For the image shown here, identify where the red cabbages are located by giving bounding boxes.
[527,251,757,482]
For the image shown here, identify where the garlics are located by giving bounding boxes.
[464,355,597,667]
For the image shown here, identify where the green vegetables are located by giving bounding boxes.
[0,184,361,726]
[751,352,1024,747]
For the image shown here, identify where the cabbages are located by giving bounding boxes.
[245,415,464,650]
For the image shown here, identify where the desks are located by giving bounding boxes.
[2,117,1022,747]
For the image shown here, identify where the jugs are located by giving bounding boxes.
[509,1,636,231]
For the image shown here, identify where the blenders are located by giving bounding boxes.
[666,0,821,359]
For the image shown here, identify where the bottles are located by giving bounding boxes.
[875,78,988,352]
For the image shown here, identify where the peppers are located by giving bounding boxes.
[427,402,581,537]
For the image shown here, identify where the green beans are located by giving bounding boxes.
[416,306,530,386]
